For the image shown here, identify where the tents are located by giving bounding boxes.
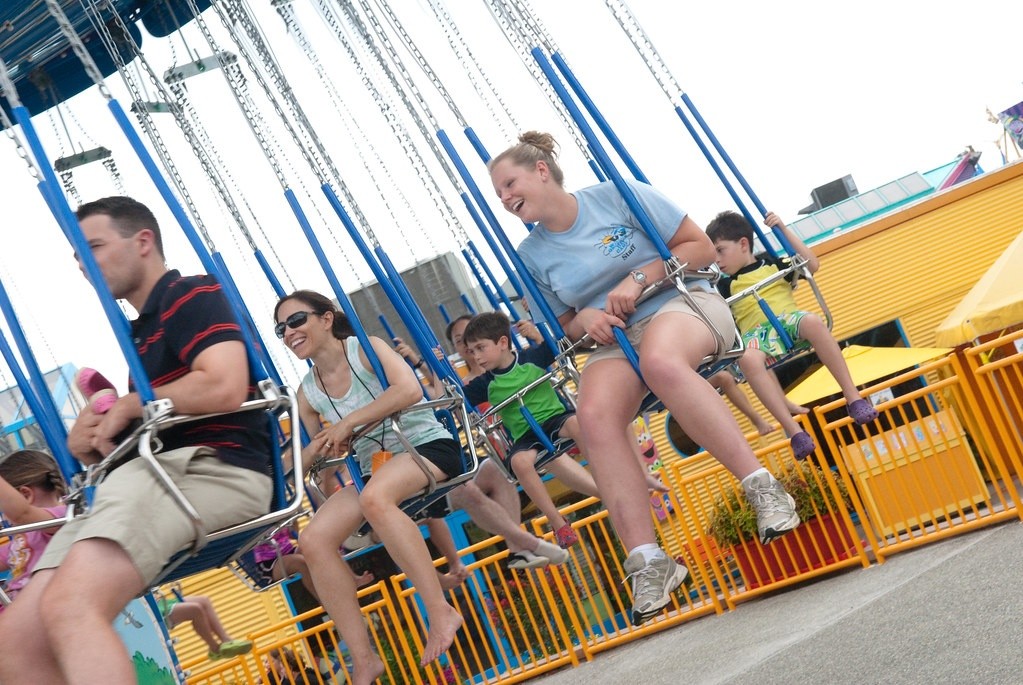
[935,230,1023,364]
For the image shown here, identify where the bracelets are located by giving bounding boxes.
[414,358,424,369]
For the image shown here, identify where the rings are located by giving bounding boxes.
[325,443,332,448]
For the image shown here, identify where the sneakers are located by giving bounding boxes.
[534,541,569,565]
[551,517,576,548]
[508,551,538,568]
[736,471,801,545]
[620,549,683,624]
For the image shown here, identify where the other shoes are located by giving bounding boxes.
[220,639,252,657]
[208,651,218,660]
[76,367,118,415]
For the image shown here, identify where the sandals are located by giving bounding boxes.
[790,433,814,459]
[849,399,878,424]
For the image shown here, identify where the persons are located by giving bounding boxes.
[485,133,799,625]
[153,593,253,661]
[430,311,592,550]
[705,209,878,461]
[707,369,809,434]
[272,292,465,685]
[257,646,330,685]
[315,457,473,590]
[0,196,274,685]
[253,523,375,611]
[0,449,84,615]
[431,296,670,500]
[391,337,568,569]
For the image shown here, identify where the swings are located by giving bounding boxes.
[2,0,835,632]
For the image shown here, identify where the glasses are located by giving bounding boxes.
[275,309,323,341]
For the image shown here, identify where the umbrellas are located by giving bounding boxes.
[784,343,955,409]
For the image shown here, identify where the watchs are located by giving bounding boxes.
[629,270,648,287]
